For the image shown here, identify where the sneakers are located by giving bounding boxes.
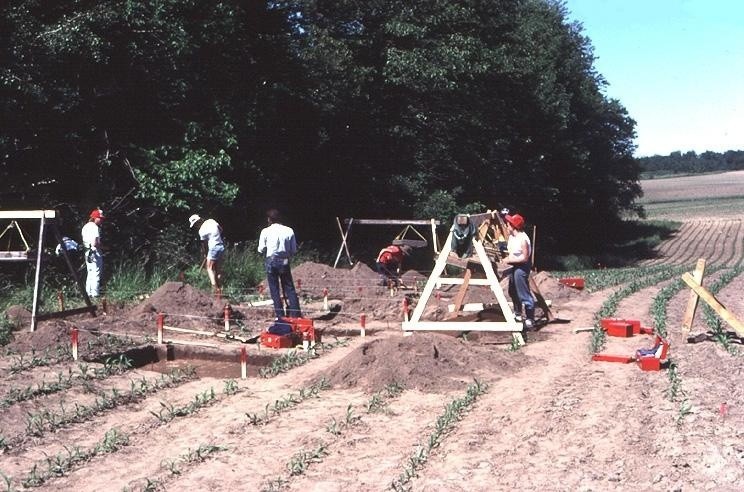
[513,313,535,328]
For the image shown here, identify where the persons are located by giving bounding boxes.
[375,243,413,289]
[186,213,224,296]
[79,208,105,298]
[255,207,302,320]
[500,213,535,328]
[54,236,79,281]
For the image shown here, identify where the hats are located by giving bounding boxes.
[504,214,524,230]
[189,213,200,228]
[89,209,105,219]
[400,245,412,255]
